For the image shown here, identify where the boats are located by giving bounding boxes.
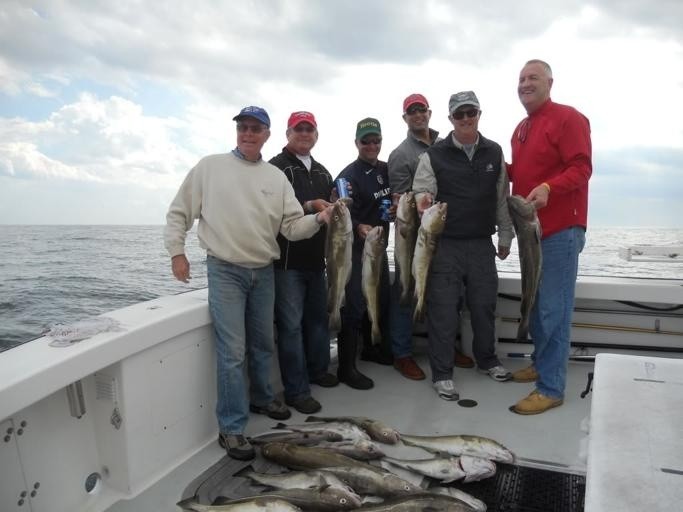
[0,268,683,512]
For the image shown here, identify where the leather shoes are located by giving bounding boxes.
[394,357,424,380]
[510,366,539,382]
[455,352,474,367]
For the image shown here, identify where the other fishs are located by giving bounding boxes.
[175,415,515,512]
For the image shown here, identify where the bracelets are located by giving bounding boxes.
[542,183,550,191]
[307,200,314,213]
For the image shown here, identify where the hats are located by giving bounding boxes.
[356,117,380,139]
[233,106,270,127]
[403,94,429,112]
[449,90,480,114]
[288,111,317,128]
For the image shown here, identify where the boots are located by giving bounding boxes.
[364,326,393,366]
[338,333,374,390]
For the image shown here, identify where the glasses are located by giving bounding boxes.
[360,138,380,144]
[237,124,263,133]
[293,125,315,133]
[450,109,480,120]
[518,119,530,145]
[406,108,427,115]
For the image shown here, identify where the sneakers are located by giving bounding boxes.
[219,432,256,461]
[308,373,340,388]
[432,379,459,401]
[478,365,512,381]
[249,403,291,421]
[513,388,564,414]
[286,395,321,414]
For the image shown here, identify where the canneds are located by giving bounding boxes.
[335,178,350,197]
[379,199,391,221]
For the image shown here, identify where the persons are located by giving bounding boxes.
[388,94,474,380]
[333,118,392,390]
[504,59,592,415]
[267,111,353,414]
[413,91,512,402]
[164,106,336,462]
